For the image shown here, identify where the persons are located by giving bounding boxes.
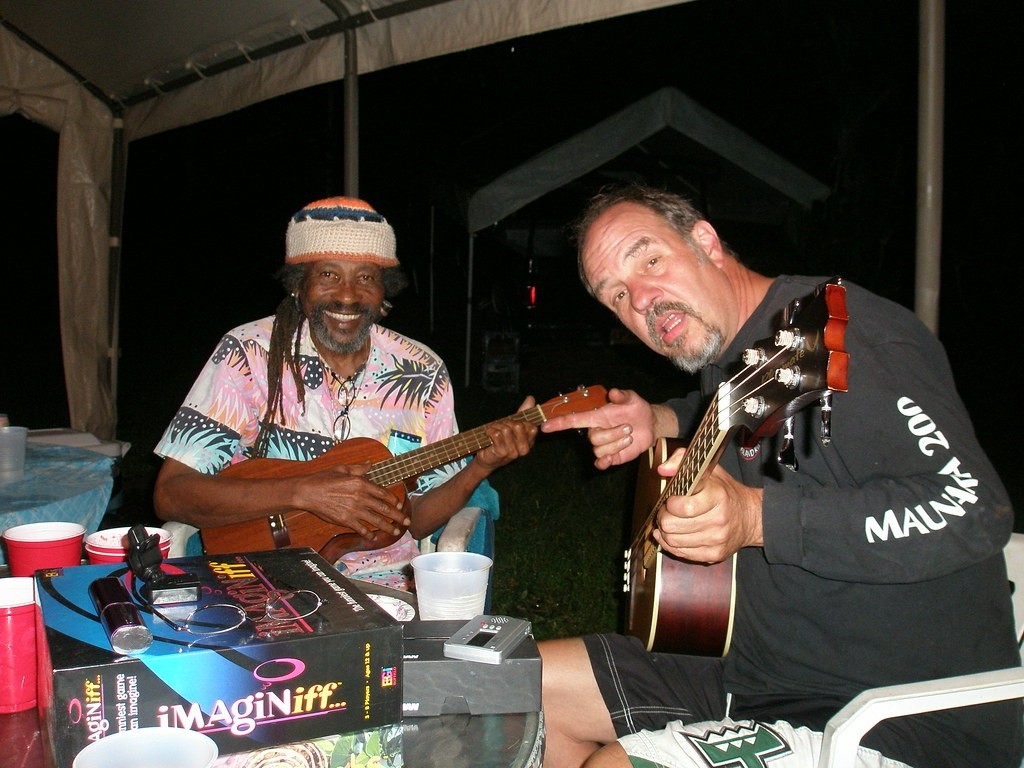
[504,185,1023,768]
[151,197,536,767]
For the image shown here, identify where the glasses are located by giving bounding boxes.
[131,550,328,635]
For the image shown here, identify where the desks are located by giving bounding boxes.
[0,441,117,564]
[1,560,548,768]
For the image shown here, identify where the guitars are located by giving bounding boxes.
[611,269,859,666]
[194,372,615,572]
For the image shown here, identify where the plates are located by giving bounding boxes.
[366,593,415,622]
[72,727,218,768]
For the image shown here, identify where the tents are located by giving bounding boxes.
[464,85,848,395]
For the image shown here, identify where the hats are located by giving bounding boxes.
[285,197,399,268]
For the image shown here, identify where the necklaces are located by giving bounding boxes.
[312,341,369,445]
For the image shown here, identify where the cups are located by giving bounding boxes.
[2,521,87,577]
[0,426,28,481]
[0,577,51,714]
[410,551,493,622]
[84,544,172,564]
[86,527,173,554]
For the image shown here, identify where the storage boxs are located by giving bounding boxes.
[398,620,542,716]
[33,548,404,767]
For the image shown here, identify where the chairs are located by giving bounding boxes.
[819,532,1024,768]
[161,454,500,615]
